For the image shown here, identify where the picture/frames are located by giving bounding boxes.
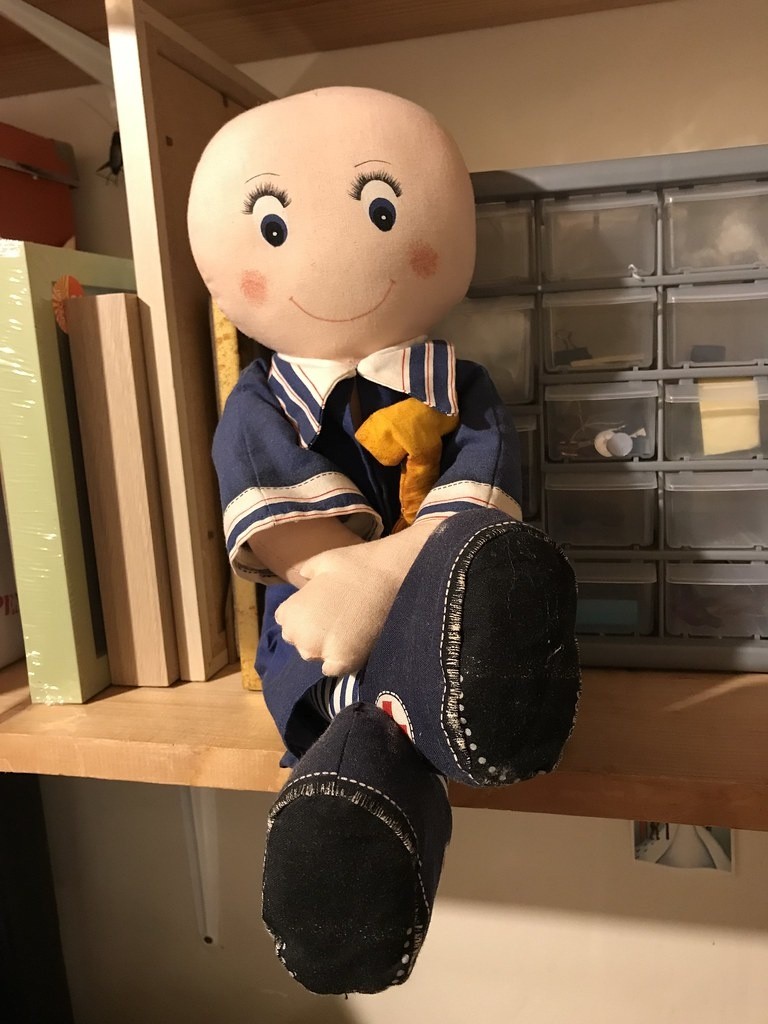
[0,240,138,706]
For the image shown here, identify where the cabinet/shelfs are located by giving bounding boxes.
[426,142,768,674]
[0,1,768,832]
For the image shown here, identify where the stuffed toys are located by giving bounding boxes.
[187,86,581,997]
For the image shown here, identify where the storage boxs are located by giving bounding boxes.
[0,121,80,248]
[426,184,768,640]
[62,291,180,689]
